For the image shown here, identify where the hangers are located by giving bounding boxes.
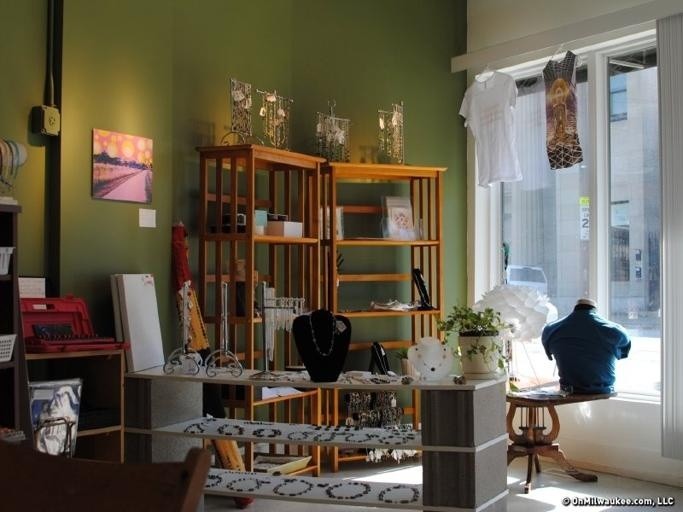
[476,64,494,81]
[550,42,568,61]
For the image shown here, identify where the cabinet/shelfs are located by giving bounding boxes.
[1,144,510,512]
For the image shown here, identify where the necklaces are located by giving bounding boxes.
[414,273,430,307]
[183,421,204,435]
[379,433,407,444]
[308,311,338,356]
[326,481,370,500]
[225,79,403,161]
[345,390,403,427]
[204,473,222,488]
[414,344,447,372]
[217,422,244,435]
[378,484,419,503]
[345,432,373,442]
[286,430,307,440]
[371,342,389,375]
[225,476,261,492]
[271,478,313,496]
[251,428,281,437]
[312,432,337,441]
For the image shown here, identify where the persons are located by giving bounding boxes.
[541,296,632,396]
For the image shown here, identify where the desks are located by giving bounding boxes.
[506,380,617,495]
